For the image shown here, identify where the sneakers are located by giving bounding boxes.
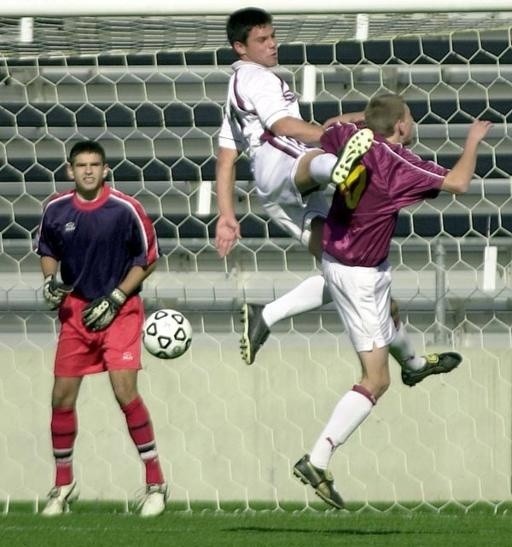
[331,128,374,184]
[41,479,80,517]
[401,352,462,385]
[294,454,344,509]
[240,303,269,366]
[139,482,166,519]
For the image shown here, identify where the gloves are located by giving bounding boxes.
[81,289,127,331]
[42,274,73,310]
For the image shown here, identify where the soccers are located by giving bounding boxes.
[141,309,192,359]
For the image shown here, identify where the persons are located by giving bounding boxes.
[217,8,372,365]
[294,91,494,508]
[37,141,171,519]
